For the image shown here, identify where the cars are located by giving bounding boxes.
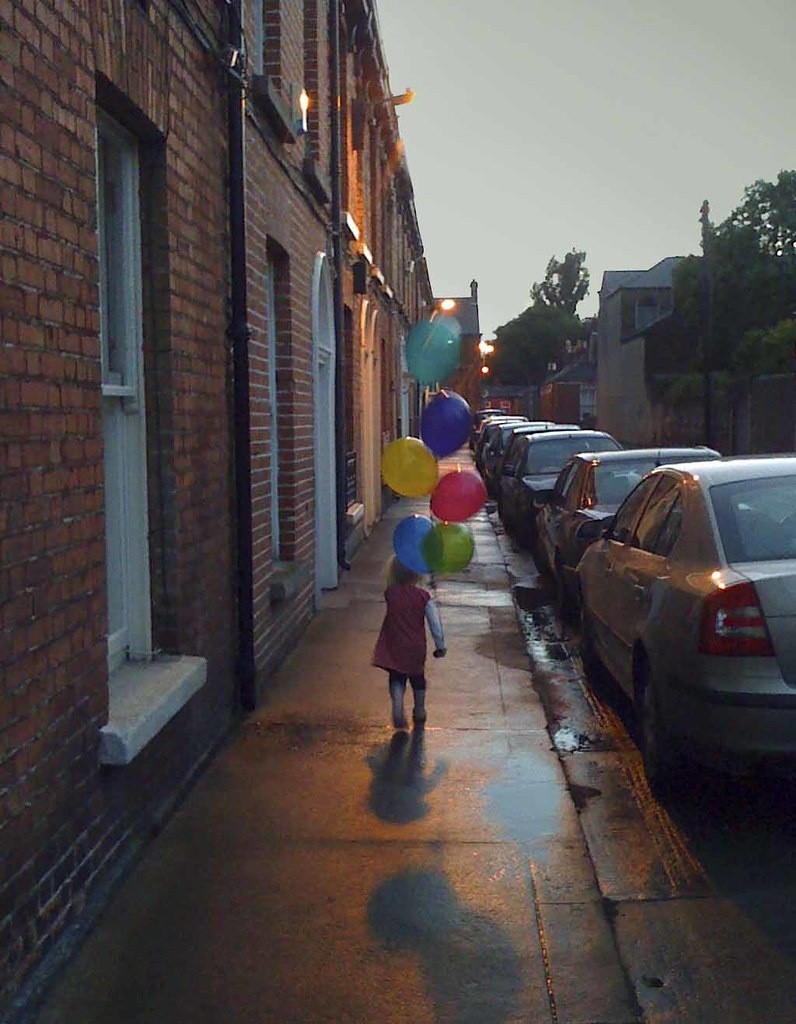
[529,443,724,631]
[469,409,624,553]
[571,451,796,804]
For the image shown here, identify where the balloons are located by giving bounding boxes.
[379,314,487,580]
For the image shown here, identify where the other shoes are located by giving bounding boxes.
[412,716,427,728]
[391,725,409,742]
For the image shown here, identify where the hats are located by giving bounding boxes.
[582,412,590,418]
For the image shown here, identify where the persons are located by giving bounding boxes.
[370,554,448,726]
